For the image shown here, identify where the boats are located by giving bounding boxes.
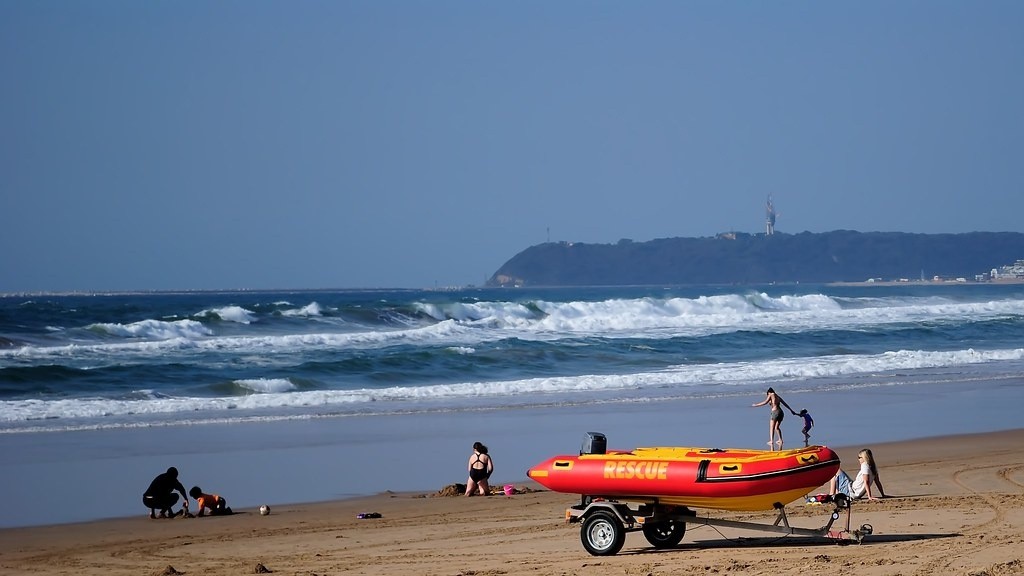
[527,430,841,511]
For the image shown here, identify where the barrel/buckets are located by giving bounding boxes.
[503,484,514,495]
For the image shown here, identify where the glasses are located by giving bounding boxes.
[858,456,862,459]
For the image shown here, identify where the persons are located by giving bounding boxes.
[189,486,233,517]
[829,449,895,502]
[752,387,794,444]
[793,408,814,442]
[464,442,493,496]
[143,467,189,519]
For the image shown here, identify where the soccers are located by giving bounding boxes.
[259,504,270,515]
